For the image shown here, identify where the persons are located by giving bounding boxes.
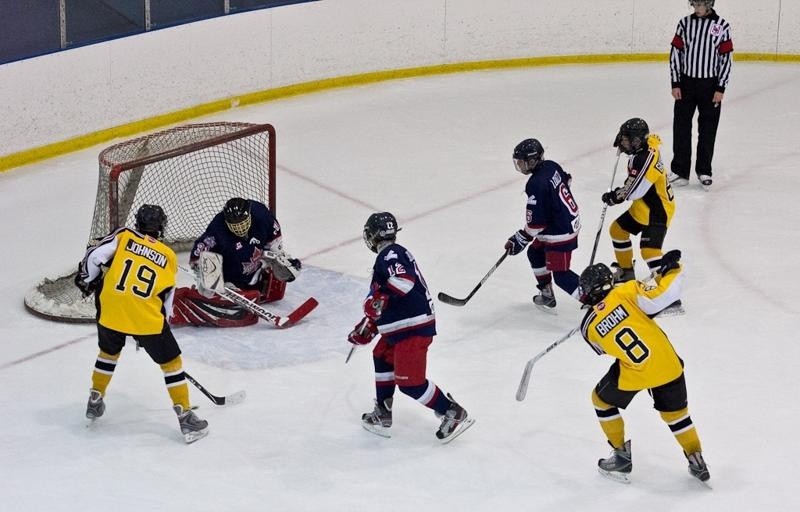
[348,211,475,442]
[190,198,301,298]
[504,137,589,314]
[578,250,710,480]
[602,118,684,314]
[667,1,733,186]
[74,204,208,443]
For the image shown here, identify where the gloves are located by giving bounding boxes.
[660,250,681,276]
[601,187,625,207]
[505,229,533,255]
[348,316,379,345]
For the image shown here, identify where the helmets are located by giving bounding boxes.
[614,118,649,155]
[578,263,615,306]
[688,1,714,18]
[224,198,252,237]
[135,204,167,238]
[513,139,544,175]
[363,212,402,254]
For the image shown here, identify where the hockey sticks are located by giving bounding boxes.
[183,371,247,405]
[515,326,580,401]
[346,317,372,361]
[178,265,319,329]
[437,248,511,306]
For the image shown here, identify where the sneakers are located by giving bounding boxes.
[533,282,556,307]
[683,450,710,481]
[610,260,636,283]
[666,171,689,186]
[362,398,393,428]
[598,440,632,473]
[697,173,712,191]
[85,389,105,419]
[173,404,208,435]
[434,393,467,440]
